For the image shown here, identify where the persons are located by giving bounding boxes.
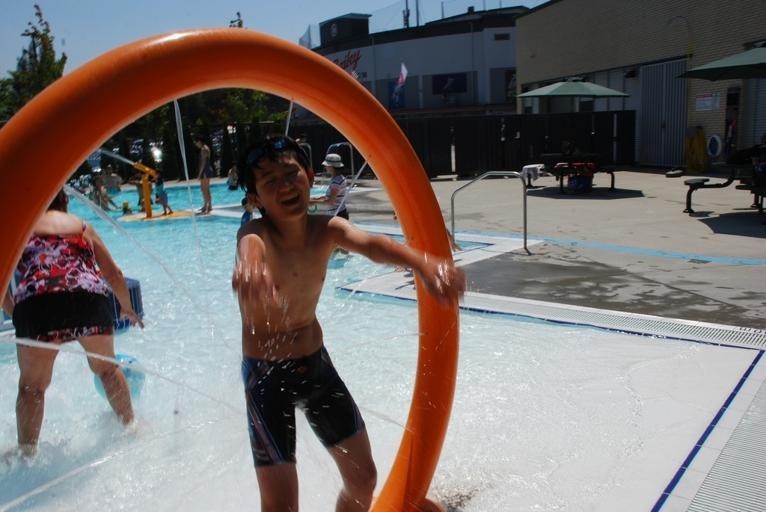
[2,186,145,458]
[231,135,466,512]
[71,134,349,220]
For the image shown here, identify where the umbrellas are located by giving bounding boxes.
[676,47,766,82]
[516,75,629,117]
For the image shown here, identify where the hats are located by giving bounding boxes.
[321,153,345,168]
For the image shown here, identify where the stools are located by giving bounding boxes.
[684,174,766,215]
[522,163,544,189]
